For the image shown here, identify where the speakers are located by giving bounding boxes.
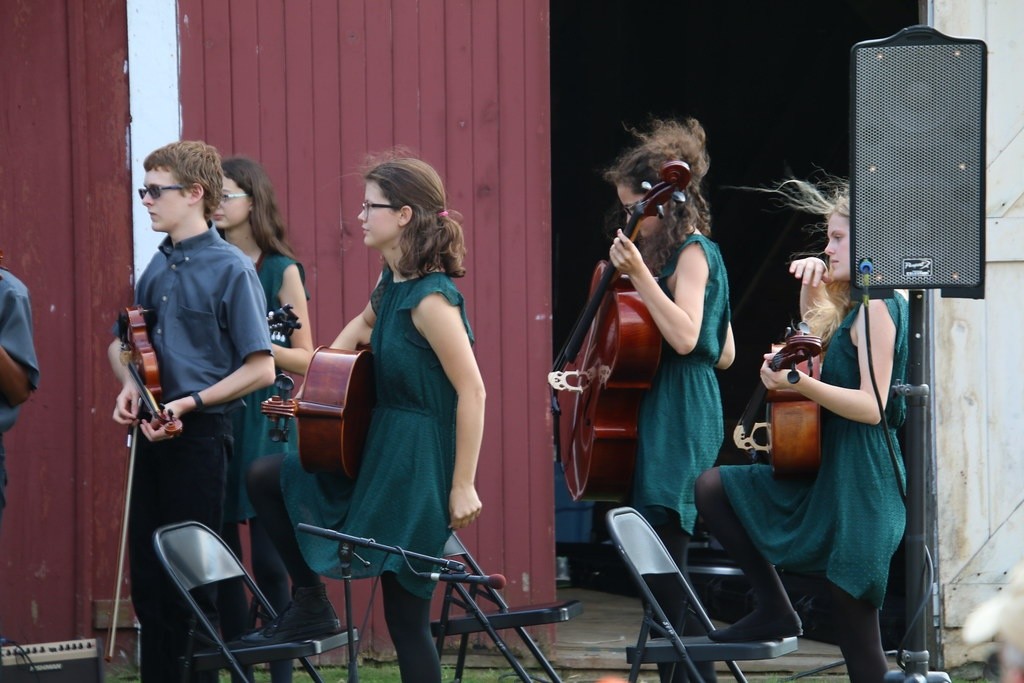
[1,637,105,683]
[849,26,987,300]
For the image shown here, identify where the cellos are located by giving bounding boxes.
[546,158,692,503]
[262,346,374,483]
[733,326,823,476]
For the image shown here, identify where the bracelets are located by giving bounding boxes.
[191,392,204,407]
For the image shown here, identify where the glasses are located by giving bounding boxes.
[219,194,251,205]
[623,199,638,216]
[362,201,401,218]
[138,185,186,200]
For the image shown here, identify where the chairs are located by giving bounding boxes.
[604,510,799,683]
[430,517,585,683]
[152,520,359,683]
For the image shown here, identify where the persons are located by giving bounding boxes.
[212,157,340,683]
[108,140,274,683]
[696,181,910,683]
[246,156,485,683]
[609,120,734,682]
[0,269,41,508]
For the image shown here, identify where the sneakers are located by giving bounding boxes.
[241,585,340,647]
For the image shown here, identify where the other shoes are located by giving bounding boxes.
[708,610,803,643]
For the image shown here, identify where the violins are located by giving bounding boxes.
[115,303,185,437]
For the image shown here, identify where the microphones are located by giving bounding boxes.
[419,572,506,591]
